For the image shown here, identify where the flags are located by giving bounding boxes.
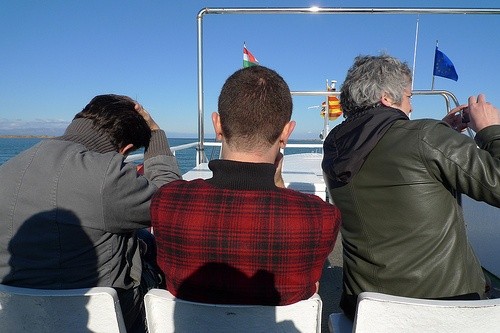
[322,85,343,120]
[243,44,259,68]
[432,47,459,82]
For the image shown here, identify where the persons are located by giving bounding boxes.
[151,65,339,307]
[0,94,181,333]
[322,54,500,326]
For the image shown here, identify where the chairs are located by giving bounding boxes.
[0,284,125,333]
[329,292,500,333]
[144,288,323,333]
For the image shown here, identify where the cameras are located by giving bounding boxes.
[461,106,470,123]
